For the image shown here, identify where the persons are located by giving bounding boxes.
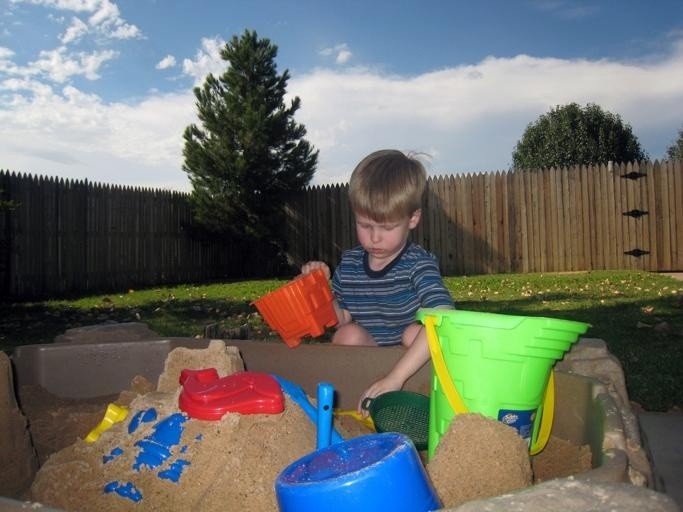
[299,147,457,418]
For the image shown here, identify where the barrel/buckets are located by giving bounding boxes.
[273,431,444,512]
[413,308,593,464]
